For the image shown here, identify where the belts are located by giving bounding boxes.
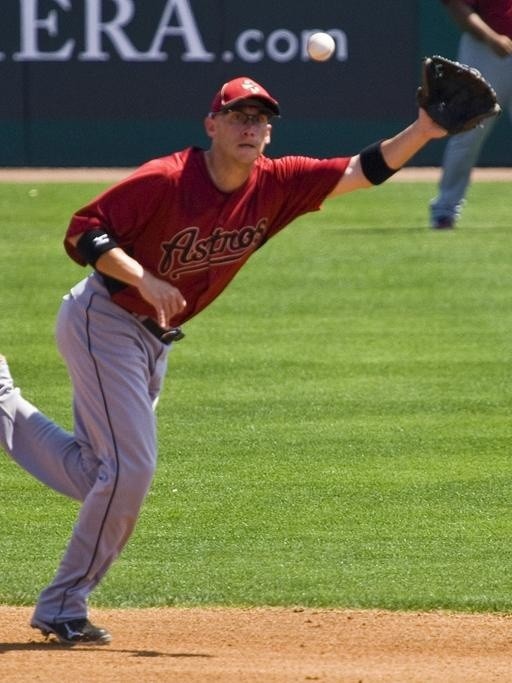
[142,317,185,345]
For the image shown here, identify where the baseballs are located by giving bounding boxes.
[307,33,335,61]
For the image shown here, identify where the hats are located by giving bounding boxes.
[212,76,281,117]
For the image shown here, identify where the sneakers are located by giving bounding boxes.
[433,217,454,228]
[31,614,112,644]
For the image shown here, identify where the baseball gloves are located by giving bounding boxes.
[416,55,502,132]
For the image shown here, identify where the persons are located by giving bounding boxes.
[426,0,511,228]
[0,55,505,651]
